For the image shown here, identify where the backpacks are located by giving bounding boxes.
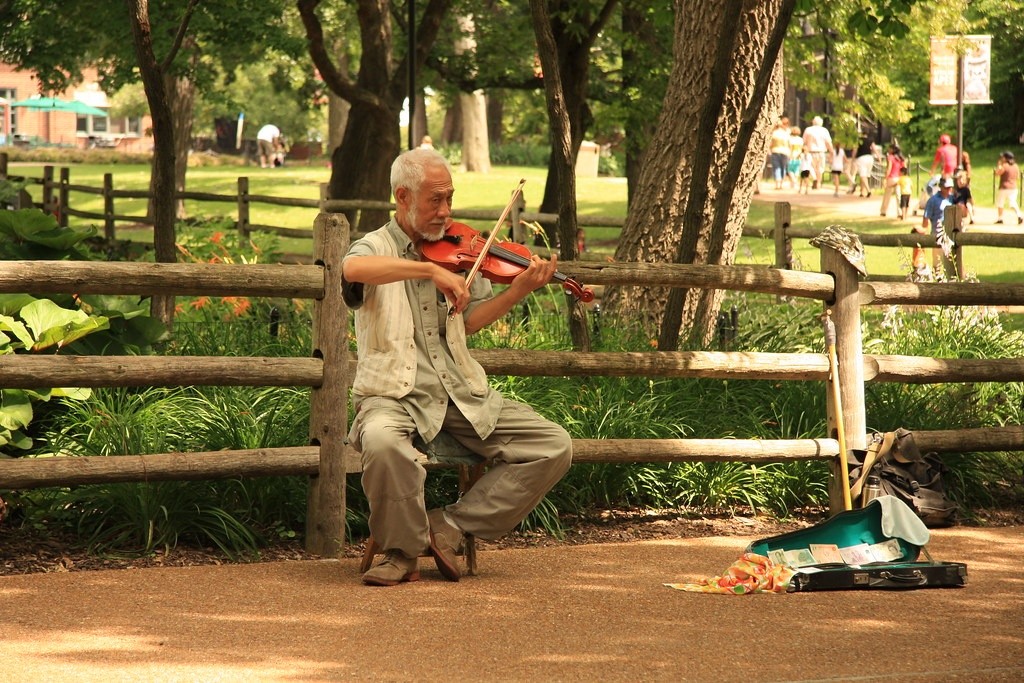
[830,428,959,527]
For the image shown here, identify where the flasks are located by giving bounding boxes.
[862,476,880,507]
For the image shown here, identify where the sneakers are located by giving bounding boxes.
[427,508,468,582]
[362,548,420,586]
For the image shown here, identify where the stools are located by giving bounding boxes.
[360,435,493,576]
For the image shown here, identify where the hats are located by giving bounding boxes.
[999,150,1014,158]
[939,177,954,188]
[940,134,951,145]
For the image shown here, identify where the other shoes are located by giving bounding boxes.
[995,220,1003,225]
[866,191,872,198]
[1018,216,1023,224]
[858,194,863,197]
[846,184,856,194]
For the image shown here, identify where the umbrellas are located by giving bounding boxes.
[10,96,68,144]
[65,100,109,146]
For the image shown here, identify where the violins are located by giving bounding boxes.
[419,216,595,303]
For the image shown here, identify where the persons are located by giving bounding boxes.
[256,124,287,169]
[420,135,433,147]
[911,132,976,282]
[768,115,880,197]
[505,220,513,242]
[50,195,60,222]
[481,230,490,239]
[338,148,574,585]
[879,136,910,221]
[993,150,1023,225]
[577,228,588,252]
